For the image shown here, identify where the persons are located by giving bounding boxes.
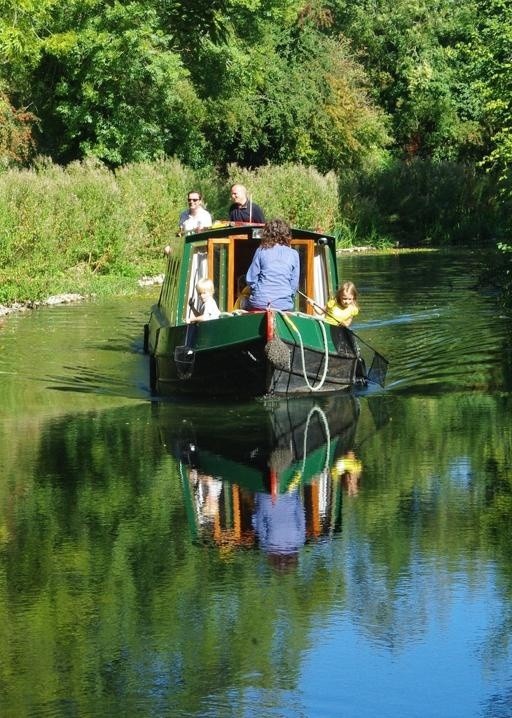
[253,493,306,573]
[179,191,212,232]
[229,185,265,225]
[185,278,221,324]
[195,476,223,543]
[306,282,359,329]
[336,450,363,499]
[239,218,300,311]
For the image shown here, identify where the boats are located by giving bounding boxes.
[153,391,362,564]
[137,223,370,395]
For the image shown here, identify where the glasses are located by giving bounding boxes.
[188,199,200,202]
